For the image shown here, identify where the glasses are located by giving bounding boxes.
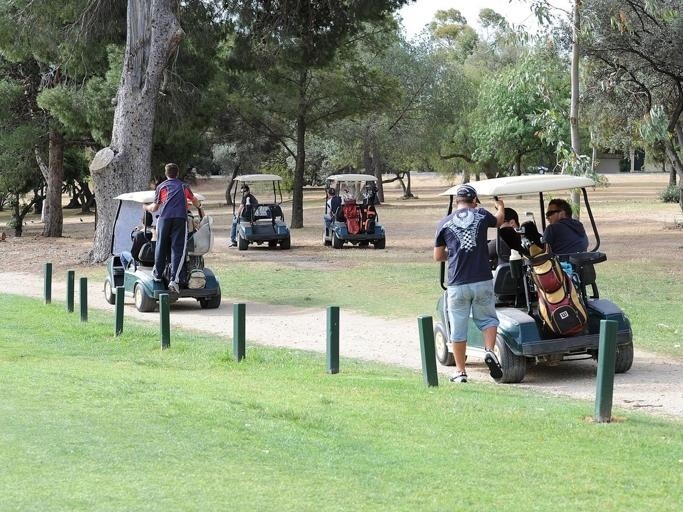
[546,210,561,216]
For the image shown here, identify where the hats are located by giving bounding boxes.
[329,188,335,194]
[456,185,480,203]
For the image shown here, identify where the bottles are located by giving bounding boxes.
[509,250,521,260]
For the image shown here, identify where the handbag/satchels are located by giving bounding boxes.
[187,269,206,289]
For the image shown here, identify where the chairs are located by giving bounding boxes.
[335,207,346,222]
[574,264,598,301]
[240,207,255,222]
[494,264,524,307]
[270,206,282,220]
[138,242,155,266]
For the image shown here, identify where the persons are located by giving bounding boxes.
[228,184,258,247]
[433,185,503,384]
[322,187,342,236]
[486,206,524,302]
[539,198,596,287]
[119,163,202,295]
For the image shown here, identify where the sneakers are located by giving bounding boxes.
[229,243,237,248]
[168,281,180,294]
[485,352,503,378]
[152,271,161,282]
[450,372,467,382]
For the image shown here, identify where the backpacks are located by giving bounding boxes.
[530,252,589,336]
